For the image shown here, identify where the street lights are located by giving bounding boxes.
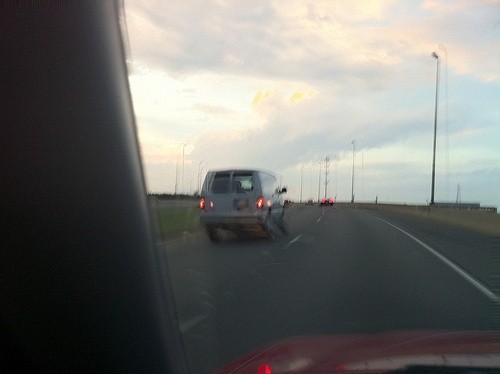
[431,51,440,207]
[298,140,356,204]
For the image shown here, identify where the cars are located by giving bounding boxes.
[284,199,334,206]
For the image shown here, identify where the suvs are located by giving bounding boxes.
[200,167,290,235]
